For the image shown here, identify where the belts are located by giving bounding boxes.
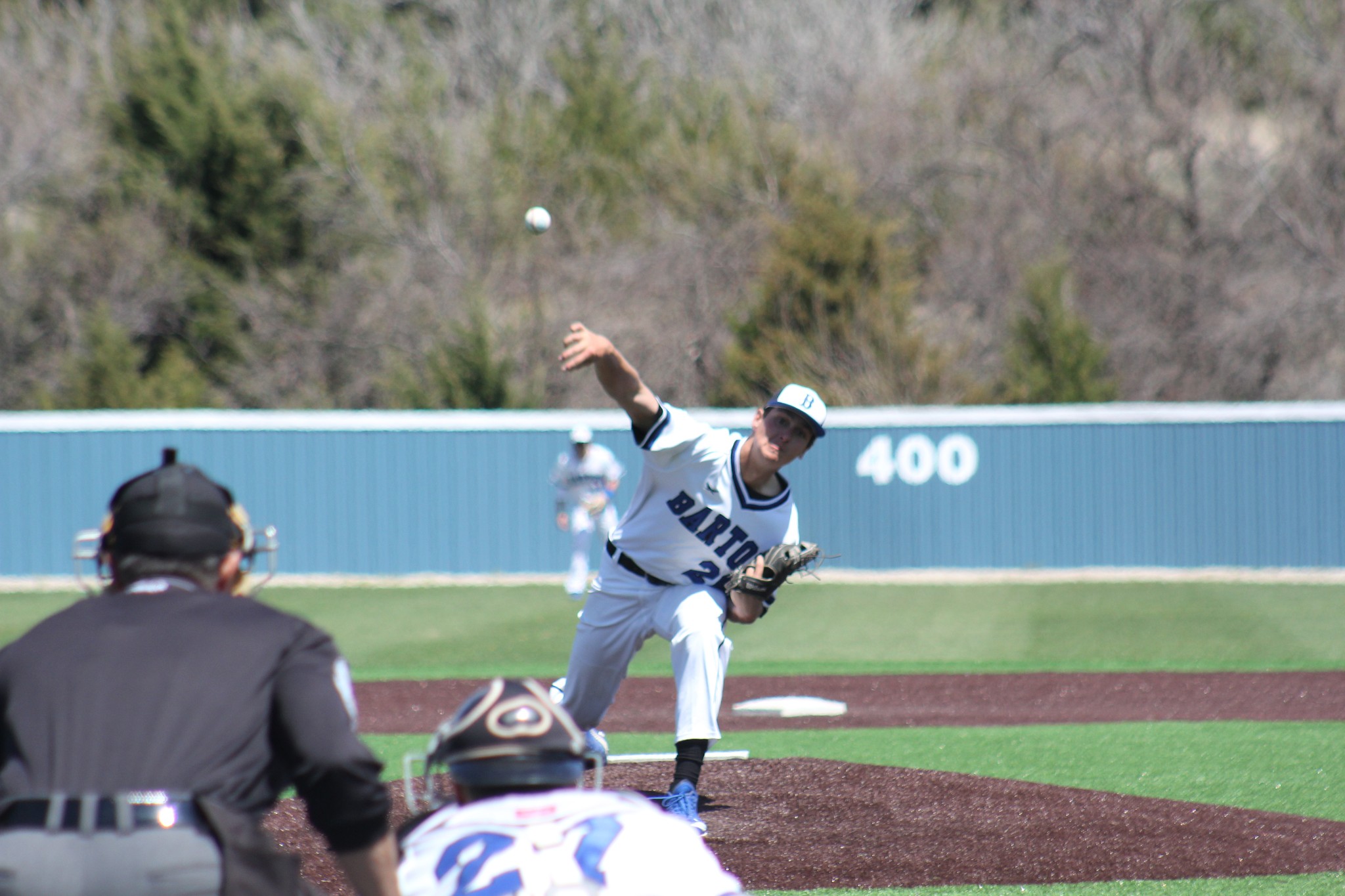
[605,533,672,586]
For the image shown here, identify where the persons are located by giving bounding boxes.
[0,447,398,896]
[556,424,622,597]
[552,320,828,837]
[390,679,741,895]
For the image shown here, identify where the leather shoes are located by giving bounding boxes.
[0,796,208,833]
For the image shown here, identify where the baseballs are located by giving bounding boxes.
[522,205,552,236]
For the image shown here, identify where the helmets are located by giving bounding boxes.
[426,679,587,786]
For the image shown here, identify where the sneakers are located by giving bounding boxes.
[647,778,708,836]
[549,678,608,764]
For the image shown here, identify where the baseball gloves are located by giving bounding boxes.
[726,541,818,601]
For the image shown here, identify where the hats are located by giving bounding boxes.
[568,424,593,444]
[101,447,255,557]
[763,384,828,439]
